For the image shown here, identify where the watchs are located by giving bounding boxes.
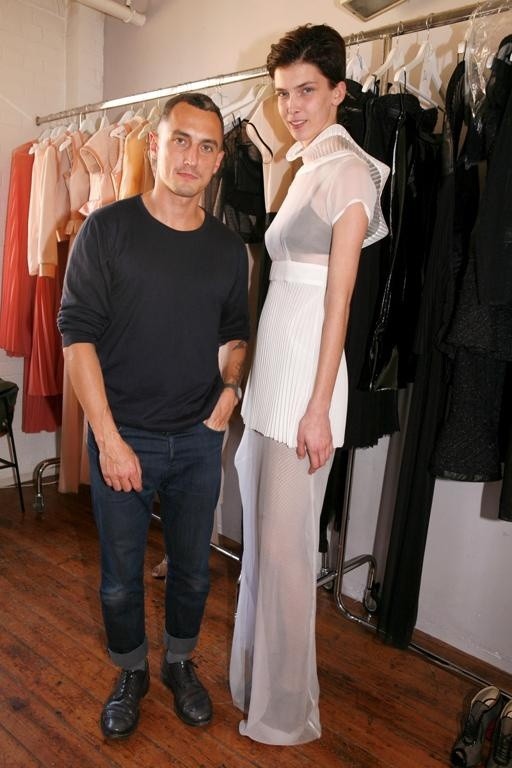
[223,383,243,401]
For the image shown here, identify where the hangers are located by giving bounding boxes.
[21,0,512,156]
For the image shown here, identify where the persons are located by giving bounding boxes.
[229,24,389,747]
[57,94,250,740]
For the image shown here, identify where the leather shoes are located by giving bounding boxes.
[160,656,213,727]
[486,699,512,768]
[151,553,168,578]
[449,685,504,768]
[101,659,150,739]
